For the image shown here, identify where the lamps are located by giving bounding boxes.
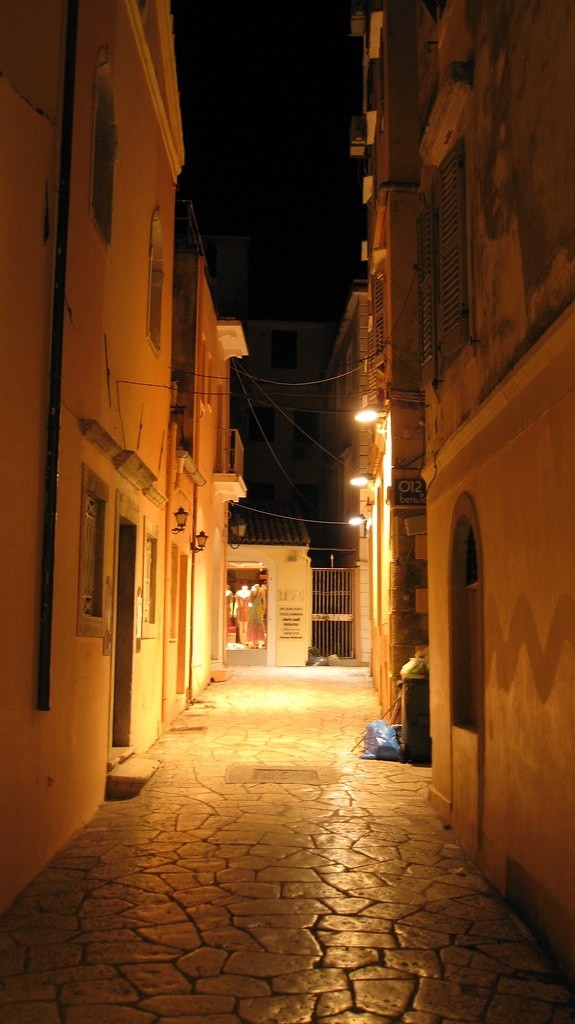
[227,512,248,549]
[355,406,386,423]
[191,530,209,552]
[173,505,189,533]
[350,472,377,487]
[349,514,369,524]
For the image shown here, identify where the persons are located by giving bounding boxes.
[226,583,268,649]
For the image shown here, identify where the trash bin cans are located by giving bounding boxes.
[398,677,432,765]
